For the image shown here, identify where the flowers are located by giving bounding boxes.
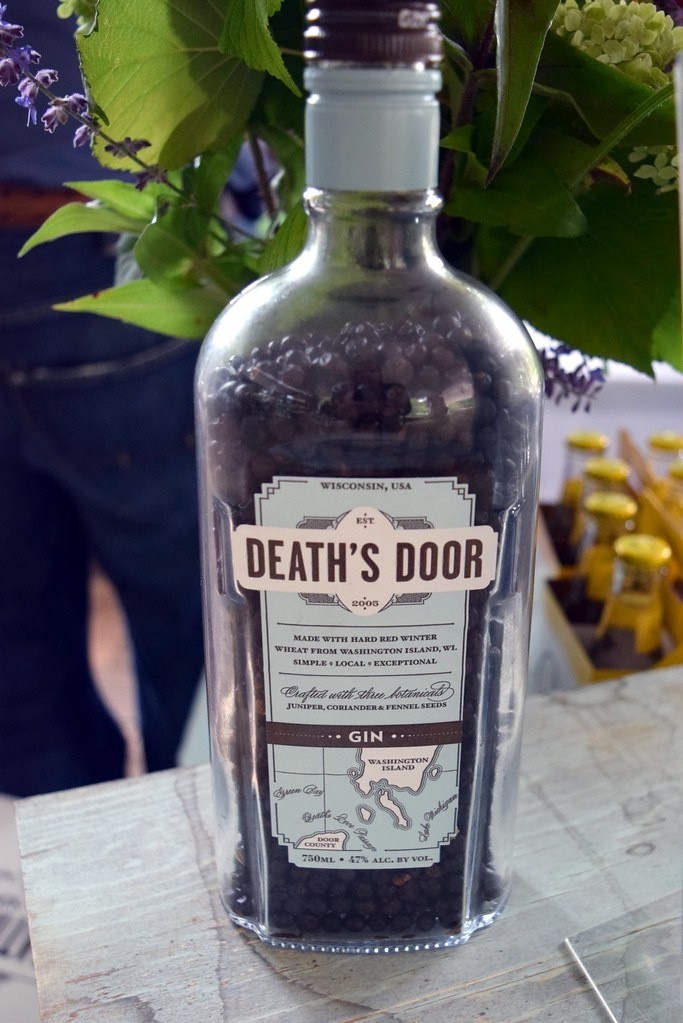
[1,0,683,345]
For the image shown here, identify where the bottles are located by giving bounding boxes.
[193,2,541,952]
[558,430,683,668]
